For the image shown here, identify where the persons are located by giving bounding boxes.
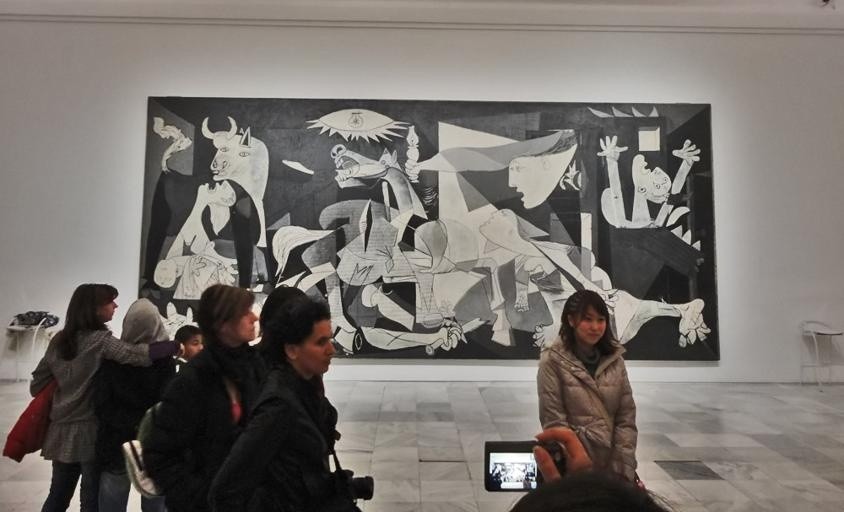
[246,284,306,375]
[30,286,185,512]
[168,326,204,376]
[143,283,261,512]
[504,426,666,511]
[91,296,174,511]
[207,297,340,511]
[535,289,637,486]
[137,321,204,511]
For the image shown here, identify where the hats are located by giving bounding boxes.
[198,284,248,331]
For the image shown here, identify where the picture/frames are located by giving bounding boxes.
[139,91,722,363]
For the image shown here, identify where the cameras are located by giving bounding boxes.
[484,440,566,492]
[330,469,374,502]
